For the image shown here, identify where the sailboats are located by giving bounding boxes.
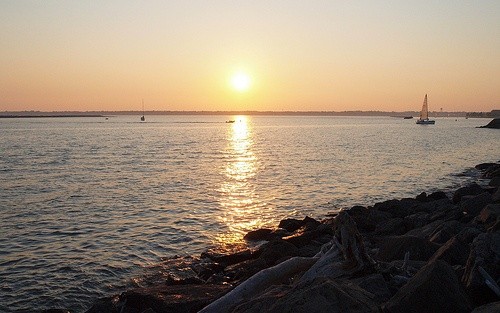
[416,94,436,125]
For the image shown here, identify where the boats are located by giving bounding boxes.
[404,116,413,119]
